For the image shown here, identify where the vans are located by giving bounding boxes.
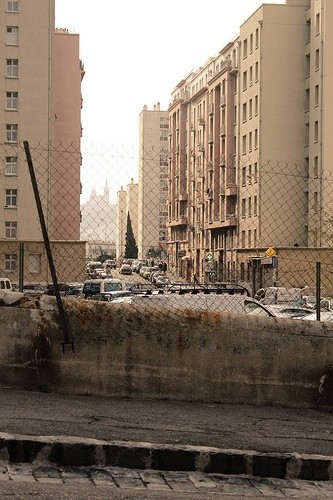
[0,278,13,292]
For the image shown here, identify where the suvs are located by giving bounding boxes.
[102,260,117,270]
[81,279,123,300]
[43,281,80,296]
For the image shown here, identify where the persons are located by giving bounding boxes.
[159,262,168,275]
[151,260,154,267]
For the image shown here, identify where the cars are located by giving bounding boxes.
[119,258,171,288]
[85,261,109,279]
[245,288,333,320]
[13,283,45,293]
